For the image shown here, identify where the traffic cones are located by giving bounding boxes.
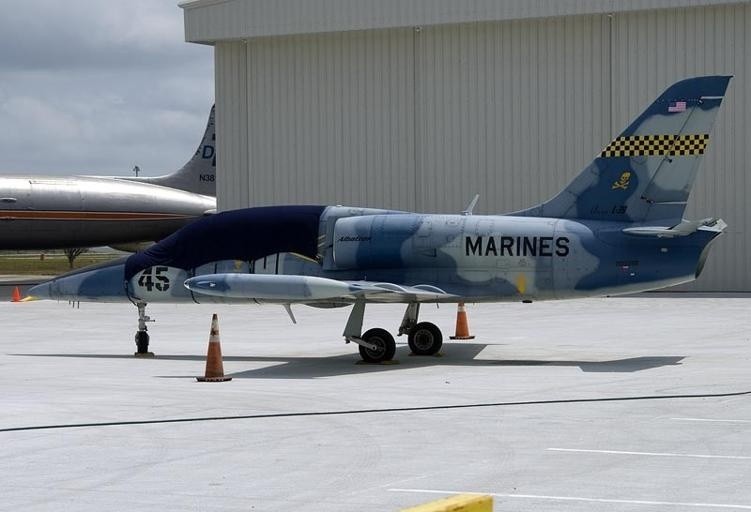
[449,302,477,340]
[196,313,233,381]
[11,284,21,302]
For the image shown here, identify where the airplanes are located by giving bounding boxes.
[0,102,217,254]
[25,73,735,365]
